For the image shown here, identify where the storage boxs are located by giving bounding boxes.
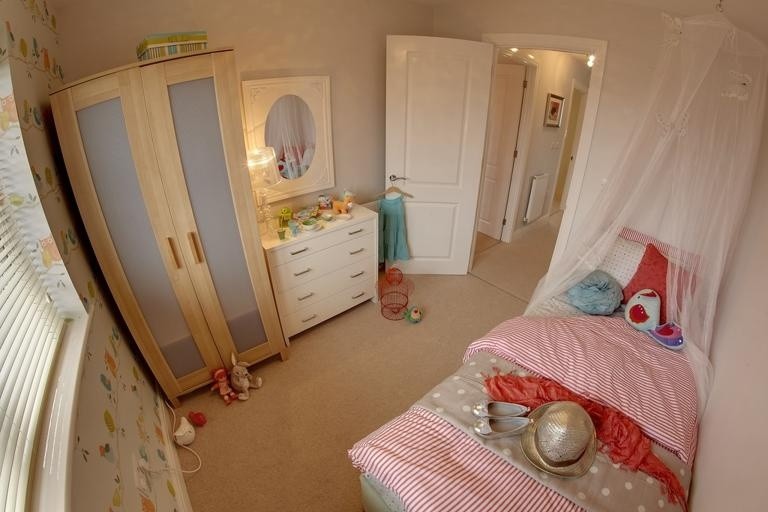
[136,29,208,62]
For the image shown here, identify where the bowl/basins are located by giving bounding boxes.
[302,221,319,230]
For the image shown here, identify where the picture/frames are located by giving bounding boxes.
[542,93,566,129]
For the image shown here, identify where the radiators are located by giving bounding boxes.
[523,173,550,225]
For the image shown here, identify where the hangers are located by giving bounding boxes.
[375,176,414,199]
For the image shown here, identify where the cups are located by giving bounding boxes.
[278,229,286,239]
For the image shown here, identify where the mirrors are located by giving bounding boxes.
[240,75,336,206]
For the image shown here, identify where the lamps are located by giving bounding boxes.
[246,146,283,239]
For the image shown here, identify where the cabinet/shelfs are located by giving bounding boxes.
[261,202,379,348]
[48,46,289,408]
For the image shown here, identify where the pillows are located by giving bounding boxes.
[598,236,699,324]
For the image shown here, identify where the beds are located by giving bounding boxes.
[355,216,713,512]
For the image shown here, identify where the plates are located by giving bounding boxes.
[300,225,323,233]
[336,214,353,221]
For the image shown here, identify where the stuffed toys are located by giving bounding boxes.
[403,304,425,323]
[332,194,353,214]
[230,361,262,401]
[211,367,237,405]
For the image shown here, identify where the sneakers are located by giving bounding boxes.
[473,417,529,439]
[471,400,528,419]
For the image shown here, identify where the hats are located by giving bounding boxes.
[518,400,598,480]
